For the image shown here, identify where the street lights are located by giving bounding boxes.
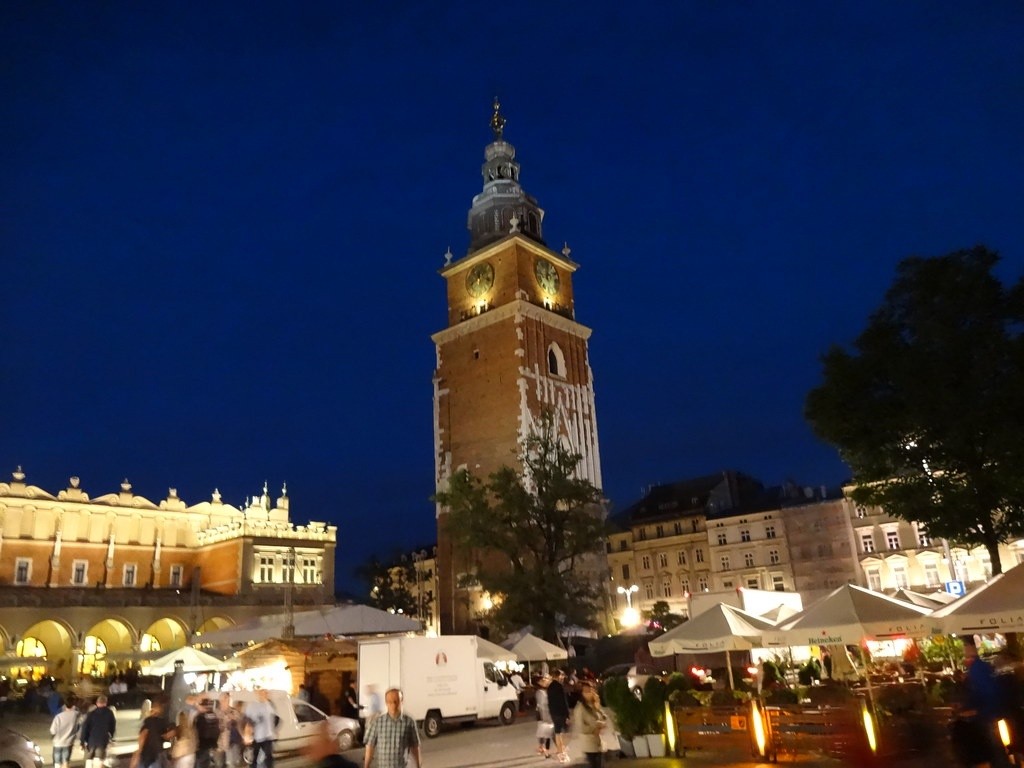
[906,440,959,580]
[617,585,639,608]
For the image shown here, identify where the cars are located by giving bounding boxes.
[0,724,46,768]
[229,690,361,761]
[597,663,667,701]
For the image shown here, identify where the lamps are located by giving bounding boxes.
[465,261,495,298]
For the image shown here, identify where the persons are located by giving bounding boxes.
[2,654,1024,767]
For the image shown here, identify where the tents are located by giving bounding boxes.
[190,603,424,648]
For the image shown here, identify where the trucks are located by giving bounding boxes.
[359,634,518,737]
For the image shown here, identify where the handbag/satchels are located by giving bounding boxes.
[536,721,555,738]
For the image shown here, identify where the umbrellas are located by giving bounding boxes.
[145,646,236,677]
[498,559,1024,695]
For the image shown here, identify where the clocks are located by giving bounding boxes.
[535,258,560,295]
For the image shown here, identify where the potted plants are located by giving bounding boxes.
[602,675,668,757]
